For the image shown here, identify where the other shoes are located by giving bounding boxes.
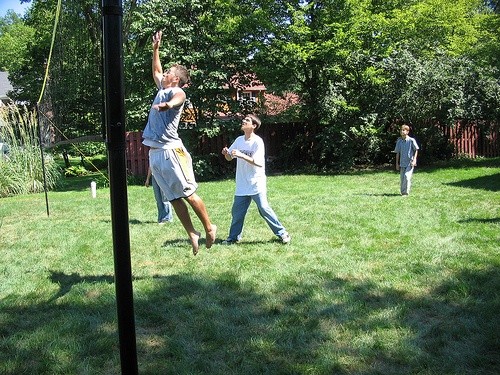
[224,238,239,245]
[280,232,290,244]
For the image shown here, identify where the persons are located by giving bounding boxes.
[145,147,173,225]
[142,31,216,255]
[395,125,419,197]
[222,115,291,244]
